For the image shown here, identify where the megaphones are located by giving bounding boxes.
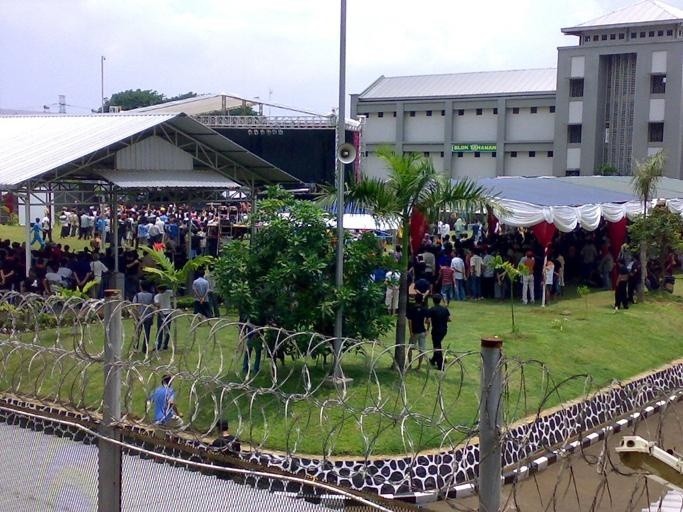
[337,143,356,164]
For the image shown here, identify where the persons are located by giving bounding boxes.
[427,294,451,370]
[149,374,185,431]
[211,418,240,481]
[405,294,430,372]
[242,321,263,374]
[266,319,285,375]
[0,193,676,322]
[131,281,154,355]
[152,286,173,351]
